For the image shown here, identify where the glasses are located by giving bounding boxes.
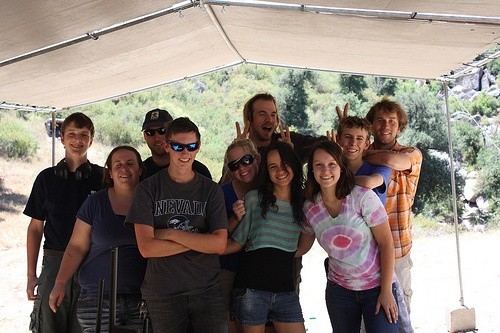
[144,127,168,136]
[167,140,199,152]
[228,154,255,172]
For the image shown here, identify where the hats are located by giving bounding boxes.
[141,109,174,131]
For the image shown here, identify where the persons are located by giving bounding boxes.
[126,117,229,333]
[227,142,306,333]
[219,137,261,238]
[49,147,147,333]
[295,139,399,333]
[23,112,113,333]
[139,109,212,182]
[219,93,307,183]
[326,116,414,333]
[336,98,423,315]
